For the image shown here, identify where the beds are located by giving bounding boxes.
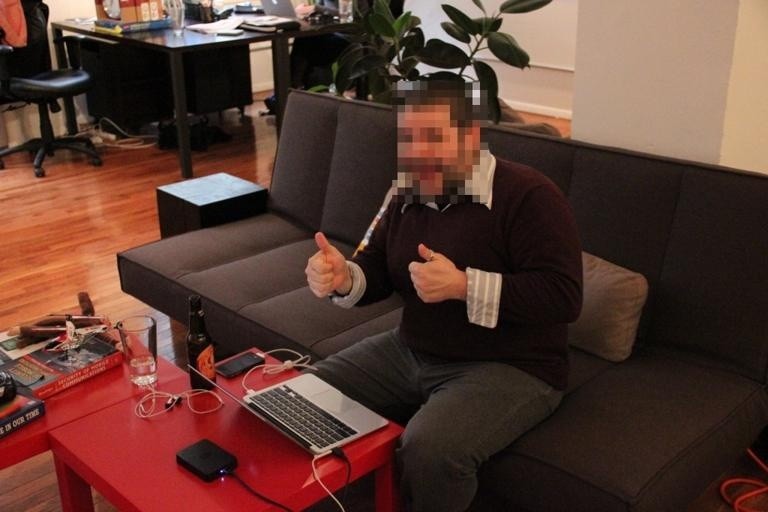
[0,306,407,512]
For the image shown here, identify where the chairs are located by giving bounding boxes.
[0,0,102,179]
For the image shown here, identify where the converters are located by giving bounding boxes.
[176,439,238,480]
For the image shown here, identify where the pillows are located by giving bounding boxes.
[566,252,650,363]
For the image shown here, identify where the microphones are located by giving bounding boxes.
[4,375,45,387]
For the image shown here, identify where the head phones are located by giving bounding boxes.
[0,371,16,407]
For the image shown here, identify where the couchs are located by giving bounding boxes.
[116,86,766,512]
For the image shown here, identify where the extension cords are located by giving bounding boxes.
[87,129,117,140]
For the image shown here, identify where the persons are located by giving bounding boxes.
[41,313,125,363]
[301,69,584,509]
[265,1,368,115]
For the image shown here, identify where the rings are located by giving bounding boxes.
[427,249,433,262]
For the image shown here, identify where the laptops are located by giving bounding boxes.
[188,361,389,460]
[261,0,307,20]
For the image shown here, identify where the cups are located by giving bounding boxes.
[201,7,215,24]
[118,315,159,386]
[170,7,186,34]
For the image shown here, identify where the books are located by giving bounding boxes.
[1,393,49,438]
[0,333,122,404]
[239,21,301,33]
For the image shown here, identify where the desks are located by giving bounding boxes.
[48,16,367,179]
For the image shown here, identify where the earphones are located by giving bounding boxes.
[173,396,182,403]
[166,398,172,408]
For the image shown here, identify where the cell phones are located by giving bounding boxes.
[215,351,266,379]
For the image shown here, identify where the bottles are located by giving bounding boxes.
[186,295,217,390]
